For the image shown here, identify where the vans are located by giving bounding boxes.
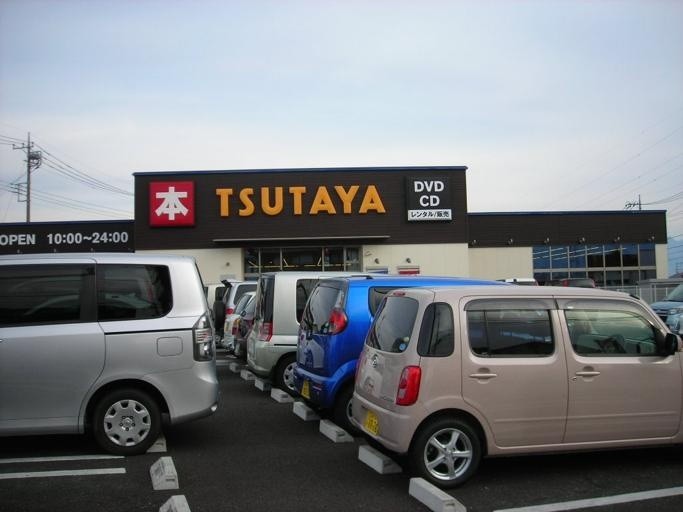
[543,277,595,288]
[202,278,256,361]
[649,285,682,336]
[244,270,388,394]
[0,253,222,460]
[498,278,538,287]
[348,284,682,491]
[292,278,507,417]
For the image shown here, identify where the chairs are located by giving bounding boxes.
[495,310,551,353]
